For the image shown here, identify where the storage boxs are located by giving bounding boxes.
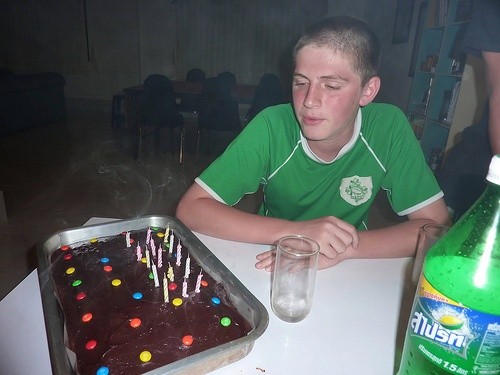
[35,215,268,375]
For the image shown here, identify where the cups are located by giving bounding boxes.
[270,234,319,324]
[410,224,449,287]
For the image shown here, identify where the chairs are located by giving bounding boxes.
[137,69,242,164]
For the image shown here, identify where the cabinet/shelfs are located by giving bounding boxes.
[405,0,487,176]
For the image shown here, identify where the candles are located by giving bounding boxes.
[125,224,203,302]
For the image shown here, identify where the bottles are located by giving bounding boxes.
[394,154,500,375]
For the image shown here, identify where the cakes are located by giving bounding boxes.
[50,226,255,375]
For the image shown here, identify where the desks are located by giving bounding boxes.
[0,217,414,375]
[123,80,292,103]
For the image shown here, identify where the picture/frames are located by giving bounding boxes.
[392,0,415,44]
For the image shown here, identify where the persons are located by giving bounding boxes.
[174,15,452,273]
[451,0,500,155]
